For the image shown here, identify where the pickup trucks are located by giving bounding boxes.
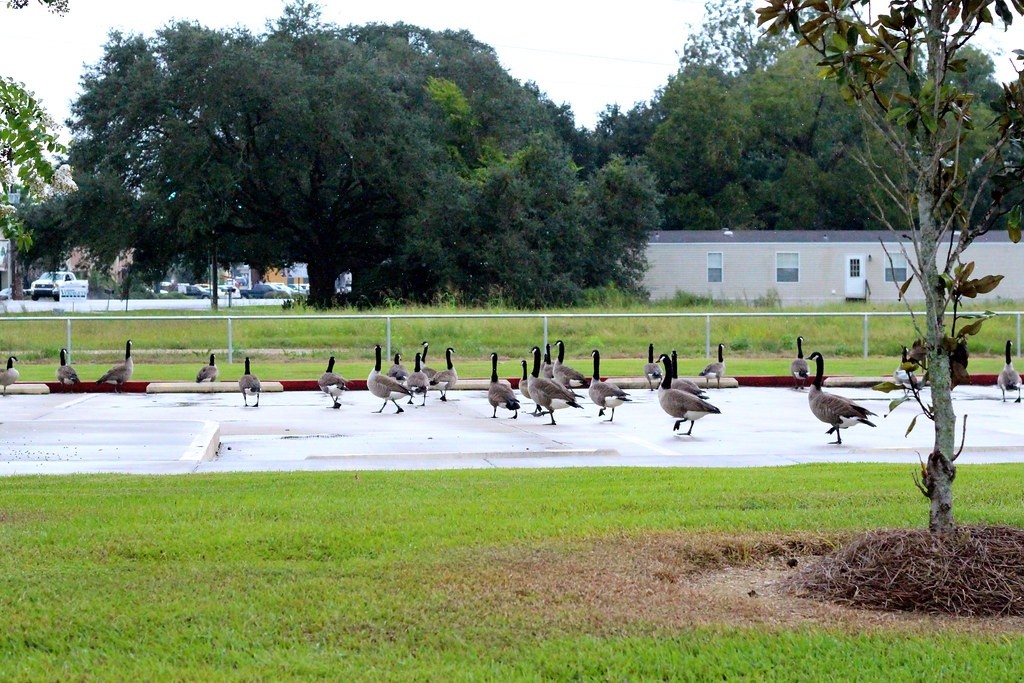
[31,272,89,302]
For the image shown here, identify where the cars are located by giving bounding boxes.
[146,281,310,299]
[0,288,30,300]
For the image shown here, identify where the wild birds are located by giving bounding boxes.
[895,345,917,401]
[791,336,811,389]
[366,344,415,413]
[318,357,354,408]
[541,339,593,390]
[56,348,82,393]
[428,347,459,402]
[407,352,431,406]
[95,338,136,393]
[388,353,410,381]
[517,346,584,424]
[488,351,520,419]
[420,342,439,385]
[997,339,1024,403]
[588,350,632,422]
[656,353,720,435]
[699,343,726,389]
[670,350,711,399]
[196,354,262,409]
[644,344,663,391]
[806,352,879,444]
[0,356,19,395]
